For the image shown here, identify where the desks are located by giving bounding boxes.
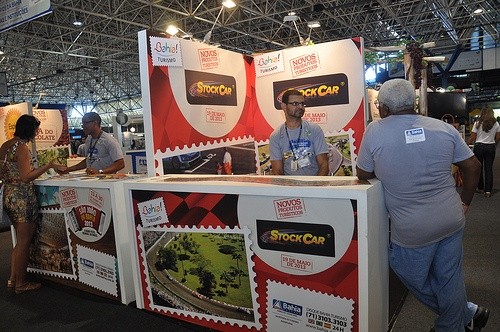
[124,174,389,332]
[10,174,136,305]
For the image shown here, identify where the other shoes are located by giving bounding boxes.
[476,189,484,195]
[486,192,492,198]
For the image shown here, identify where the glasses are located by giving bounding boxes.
[374,100,383,109]
[285,102,307,107]
[80,120,95,126]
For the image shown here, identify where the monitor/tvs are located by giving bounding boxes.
[428,92,468,123]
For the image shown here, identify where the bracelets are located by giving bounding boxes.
[462,201,466,205]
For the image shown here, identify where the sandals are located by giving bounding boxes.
[7,280,16,291]
[15,280,43,294]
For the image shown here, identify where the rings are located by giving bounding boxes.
[91,170,92,171]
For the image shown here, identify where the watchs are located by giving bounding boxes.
[98,169,104,174]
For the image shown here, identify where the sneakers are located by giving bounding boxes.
[429,327,435,332]
[465,307,490,332]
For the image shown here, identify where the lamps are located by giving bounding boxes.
[308,21,320,28]
[283,16,298,21]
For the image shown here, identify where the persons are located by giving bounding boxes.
[466,106,500,198]
[58,112,125,174]
[0,114,67,295]
[269,89,330,176]
[71,137,86,158]
[356,79,491,332]
